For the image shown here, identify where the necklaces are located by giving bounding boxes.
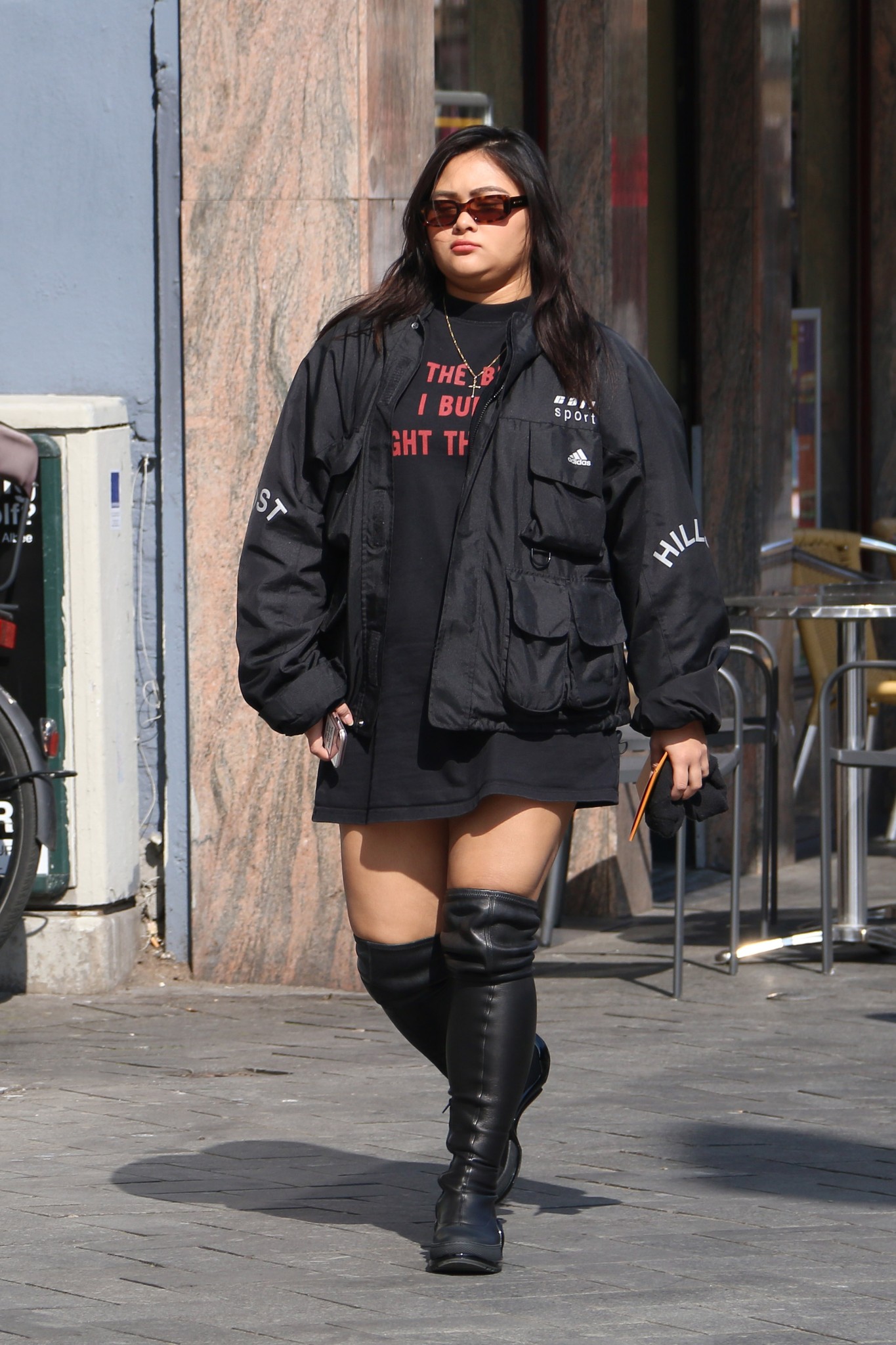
[439,292,505,398]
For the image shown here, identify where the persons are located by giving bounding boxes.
[237,126,733,1273]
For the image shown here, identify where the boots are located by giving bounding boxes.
[426,887,542,1273]
[354,934,551,1203]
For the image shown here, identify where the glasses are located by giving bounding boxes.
[417,194,529,227]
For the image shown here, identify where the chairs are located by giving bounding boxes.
[540,629,895,1000]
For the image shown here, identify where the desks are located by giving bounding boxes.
[714,583,895,965]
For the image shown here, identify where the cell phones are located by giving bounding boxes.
[322,708,347,767]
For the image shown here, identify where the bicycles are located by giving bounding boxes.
[0,418,78,952]
